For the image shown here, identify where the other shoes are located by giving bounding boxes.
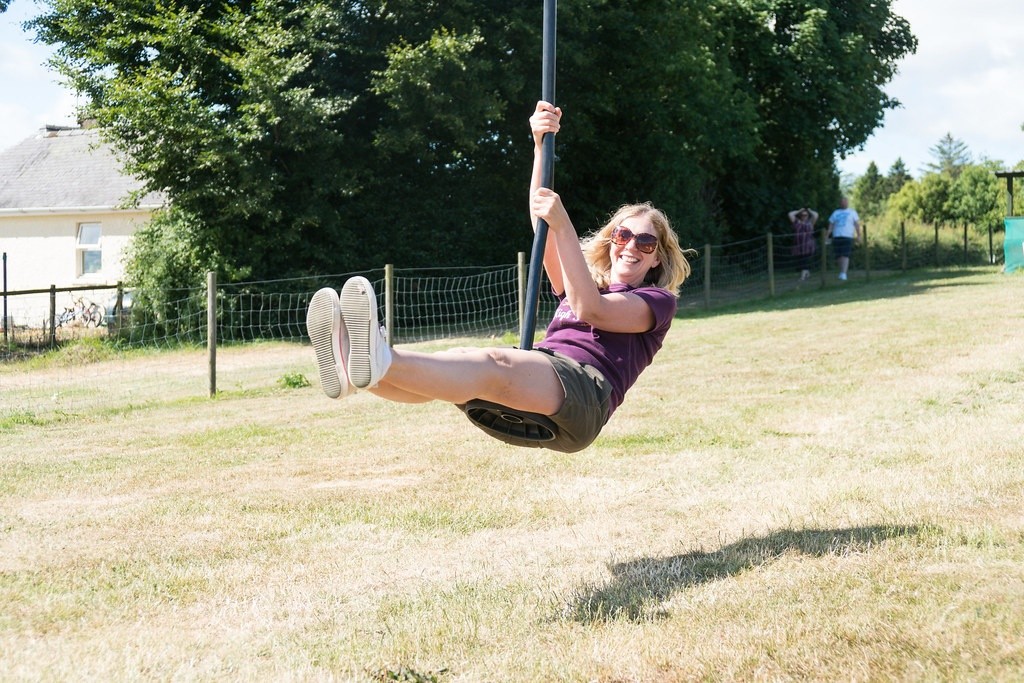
[839,272,847,280]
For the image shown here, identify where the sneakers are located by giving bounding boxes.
[339,276,393,391]
[307,287,351,400]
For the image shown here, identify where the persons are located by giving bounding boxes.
[305,101,698,453]
[788,208,819,283]
[826,195,861,281]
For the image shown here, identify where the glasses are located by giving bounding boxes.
[609,225,659,253]
[800,214,808,217]
